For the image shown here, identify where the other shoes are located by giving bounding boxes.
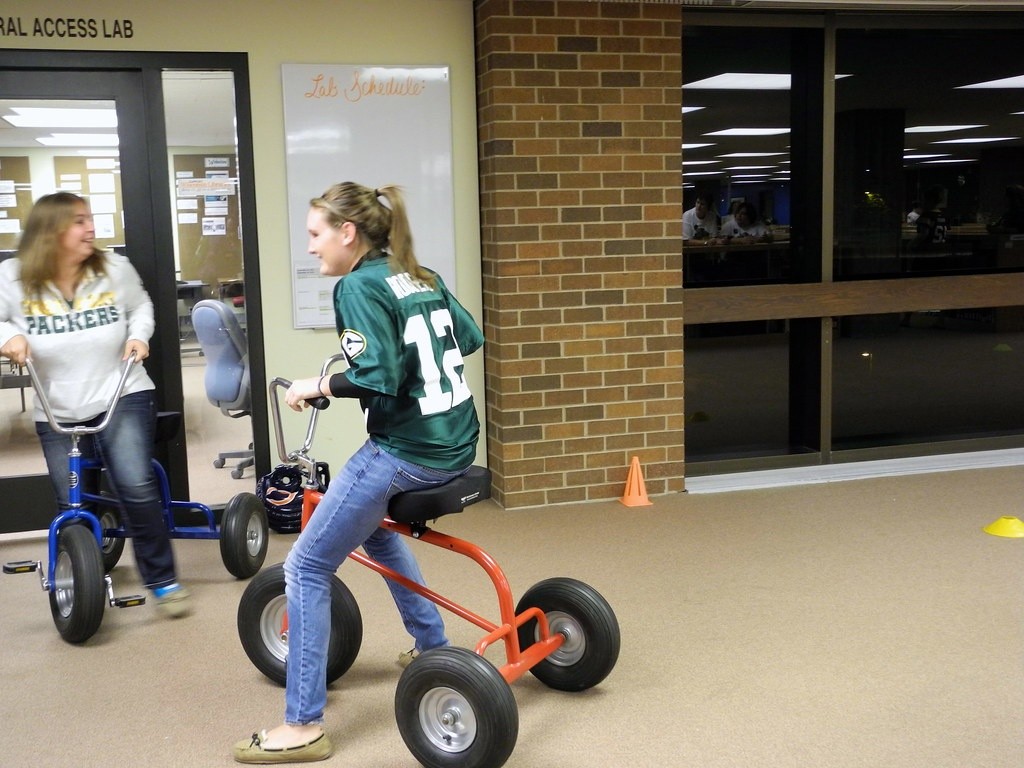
[233,729,333,764]
[399,648,419,668]
[148,585,193,617]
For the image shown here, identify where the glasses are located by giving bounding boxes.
[735,210,745,215]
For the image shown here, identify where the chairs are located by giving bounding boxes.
[191,299,255,479]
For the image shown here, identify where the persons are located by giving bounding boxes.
[896,182,1024,330]
[225,181,488,763]
[0,193,196,623]
[719,202,776,244]
[683,189,729,246]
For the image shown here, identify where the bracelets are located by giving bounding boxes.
[704,239,709,245]
[317,376,325,396]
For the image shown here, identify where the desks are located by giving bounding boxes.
[683,224,990,338]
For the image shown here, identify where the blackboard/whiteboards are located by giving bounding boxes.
[281,62,456,332]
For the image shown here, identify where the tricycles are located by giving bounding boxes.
[238,354,621,768]
[3,351,270,644]
